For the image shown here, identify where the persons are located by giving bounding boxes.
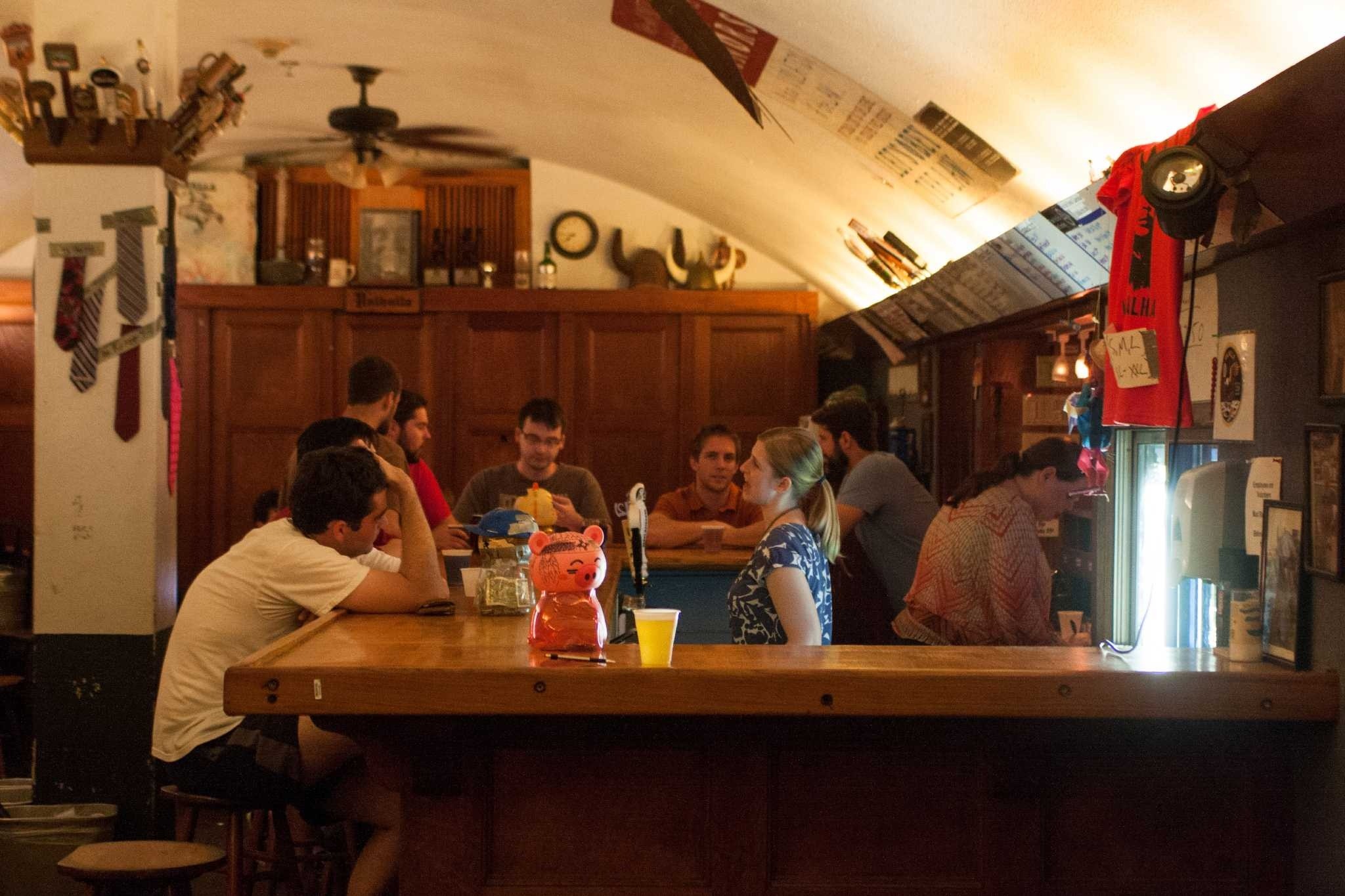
[807,400,946,601]
[641,422,776,551]
[379,389,468,572]
[908,435,1096,646]
[152,445,449,895]
[272,352,410,547]
[266,418,408,567]
[445,398,615,546]
[729,429,844,646]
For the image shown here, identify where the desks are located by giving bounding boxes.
[222,532,1342,896]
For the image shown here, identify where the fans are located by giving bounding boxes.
[194,66,513,160]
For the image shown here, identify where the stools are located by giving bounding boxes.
[60,837,225,894]
[164,782,293,896]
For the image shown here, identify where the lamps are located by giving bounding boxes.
[326,139,409,190]
[1138,123,1249,244]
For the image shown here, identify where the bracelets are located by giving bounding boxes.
[578,514,608,543]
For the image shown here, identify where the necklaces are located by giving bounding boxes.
[751,502,801,557]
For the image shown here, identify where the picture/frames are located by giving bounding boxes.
[1302,422,1345,581]
[1260,497,1313,672]
[1314,269,1345,407]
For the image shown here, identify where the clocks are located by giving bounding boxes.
[550,211,599,258]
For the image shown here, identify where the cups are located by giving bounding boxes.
[326,257,355,287]
[1058,610,1085,642]
[634,606,682,667]
[305,237,325,287]
[702,524,725,554]
[1229,588,1263,663]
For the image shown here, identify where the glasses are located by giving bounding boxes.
[521,430,564,447]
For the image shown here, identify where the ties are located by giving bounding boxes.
[53,256,87,351]
[115,226,149,324]
[68,286,106,394]
[113,323,142,442]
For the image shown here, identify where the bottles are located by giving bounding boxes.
[836,217,930,288]
[537,241,556,289]
[424,224,451,285]
[453,227,482,287]
